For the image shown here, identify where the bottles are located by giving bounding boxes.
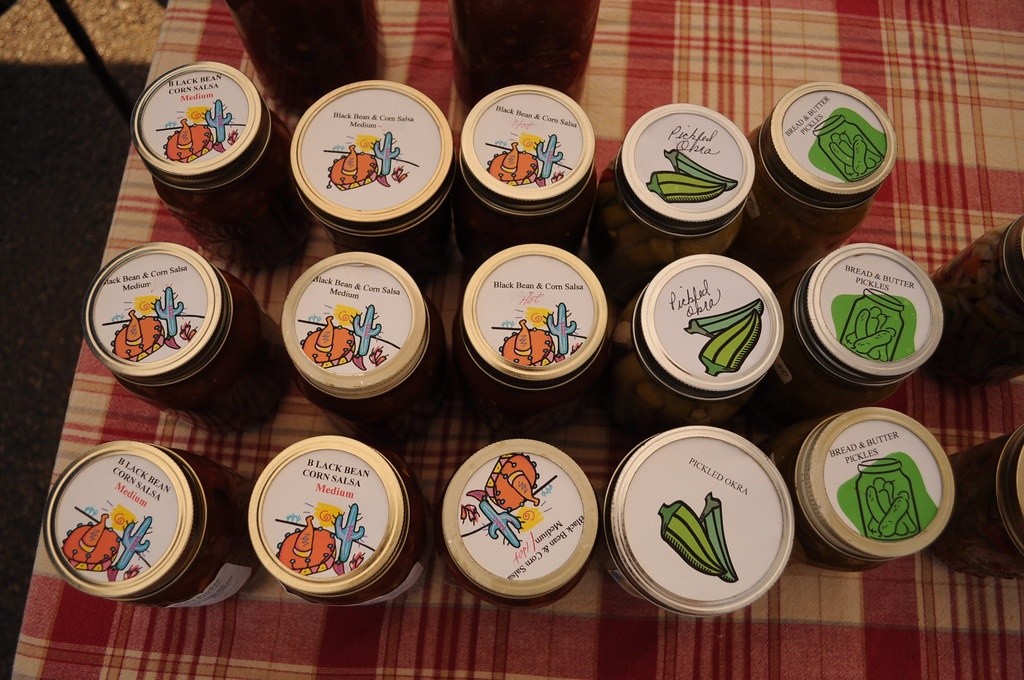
[758,403,956,573]
[607,241,944,430]
[245,433,435,608]
[128,58,309,274]
[280,251,448,450]
[587,80,898,304]
[451,242,607,438]
[287,77,601,286]
[596,424,798,618]
[42,439,255,613]
[930,423,1024,579]
[927,215,1024,391]
[79,240,291,436]
[433,436,602,611]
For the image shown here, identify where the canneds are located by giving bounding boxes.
[40,0,1024,617]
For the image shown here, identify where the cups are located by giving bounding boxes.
[222,0,388,116]
[447,0,603,111]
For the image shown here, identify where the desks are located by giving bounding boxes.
[9,0,1024,680]
